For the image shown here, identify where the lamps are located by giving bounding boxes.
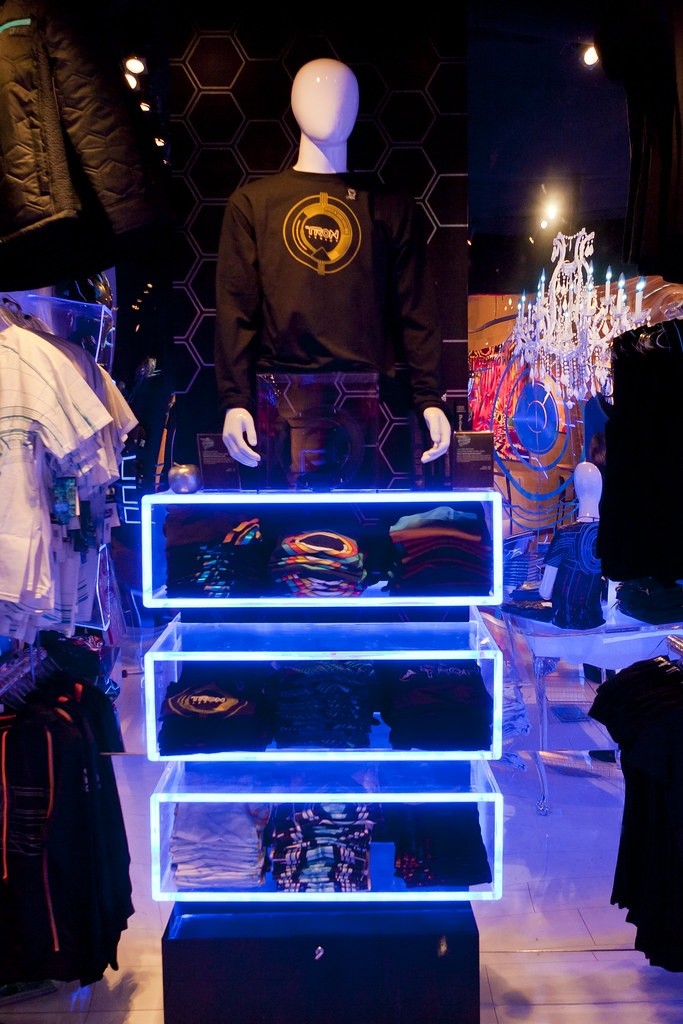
[513,227,650,410]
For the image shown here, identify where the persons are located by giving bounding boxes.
[210,58,456,486]
[536,458,618,632]
